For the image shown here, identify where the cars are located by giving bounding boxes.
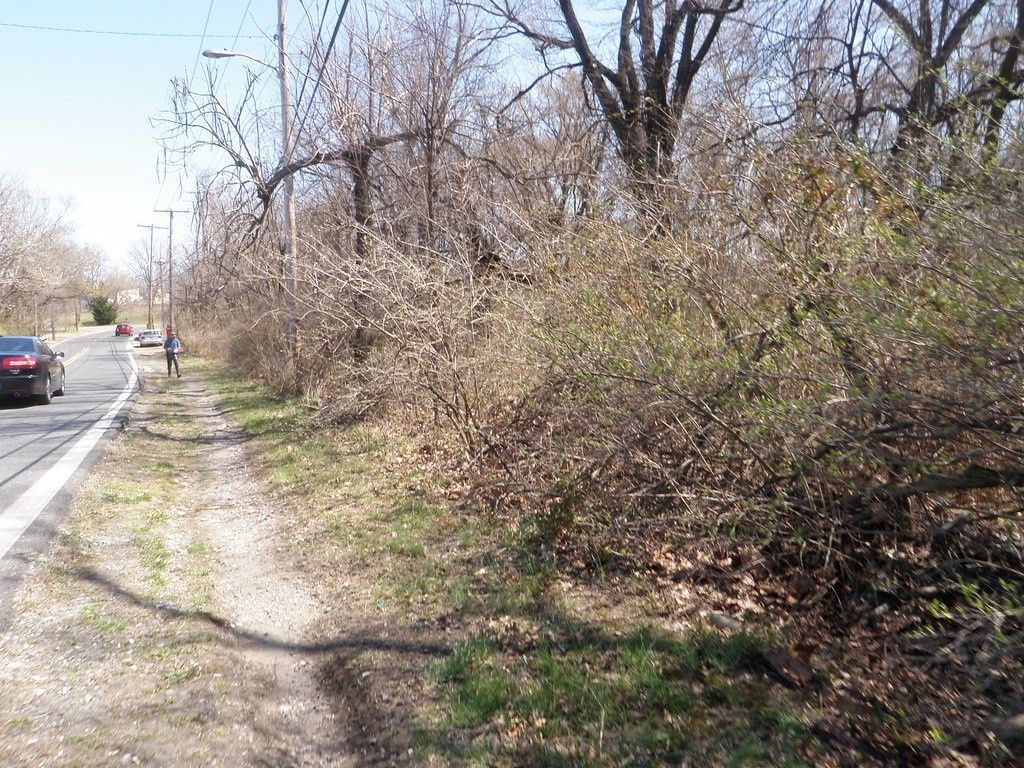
[0,335,66,405]
[139,329,163,346]
[115,324,134,336]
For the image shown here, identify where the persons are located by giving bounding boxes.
[163,332,182,379]
